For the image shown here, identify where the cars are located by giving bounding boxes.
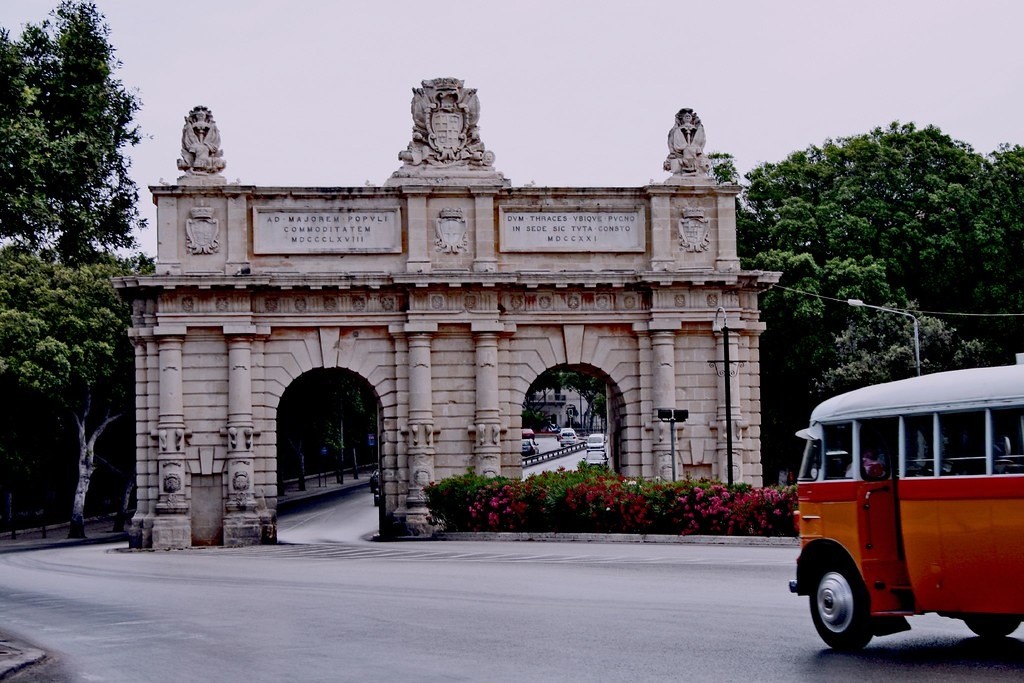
[368,469,380,508]
[582,450,609,470]
[521,428,539,458]
[556,427,580,447]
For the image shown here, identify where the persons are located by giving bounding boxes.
[847,432,1011,478]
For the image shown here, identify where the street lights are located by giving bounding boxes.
[711,304,733,488]
[848,296,921,378]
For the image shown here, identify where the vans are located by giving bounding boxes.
[788,362,1024,653]
[584,432,607,454]
